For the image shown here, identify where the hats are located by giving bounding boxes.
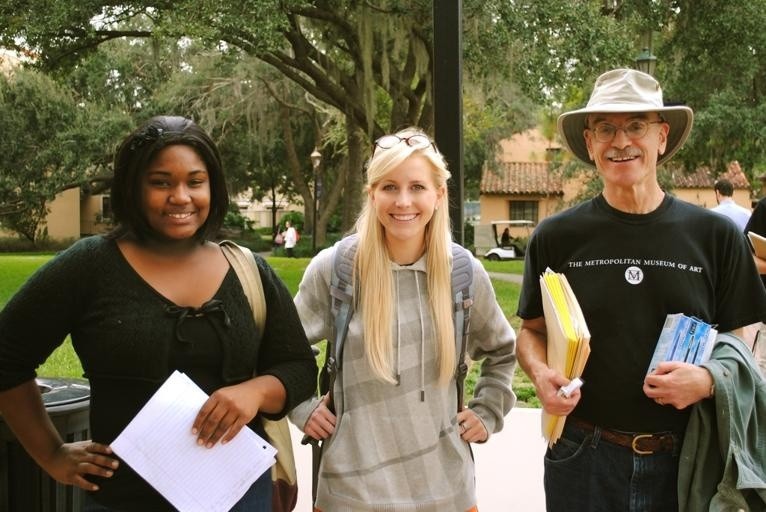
[557,68,694,170]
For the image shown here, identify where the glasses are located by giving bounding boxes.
[586,119,663,143]
[370,134,437,157]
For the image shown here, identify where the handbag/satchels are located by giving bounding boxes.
[261,415,298,512]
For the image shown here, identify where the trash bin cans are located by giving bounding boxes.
[0,374,94,512]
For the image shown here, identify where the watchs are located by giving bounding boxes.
[710,383,716,396]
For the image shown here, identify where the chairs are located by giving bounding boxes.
[493,224,514,249]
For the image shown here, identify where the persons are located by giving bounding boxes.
[516,68,766,512]
[501,227,525,256]
[710,179,752,232]
[288,127,517,512]
[0,114,319,512]
[281,220,297,257]
[743,196,766,288]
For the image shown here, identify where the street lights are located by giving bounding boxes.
[309,148,322,250]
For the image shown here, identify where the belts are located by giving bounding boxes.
[572,418,682,454]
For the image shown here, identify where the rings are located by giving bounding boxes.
[656,398,663,405]
[460,422,468,435]
